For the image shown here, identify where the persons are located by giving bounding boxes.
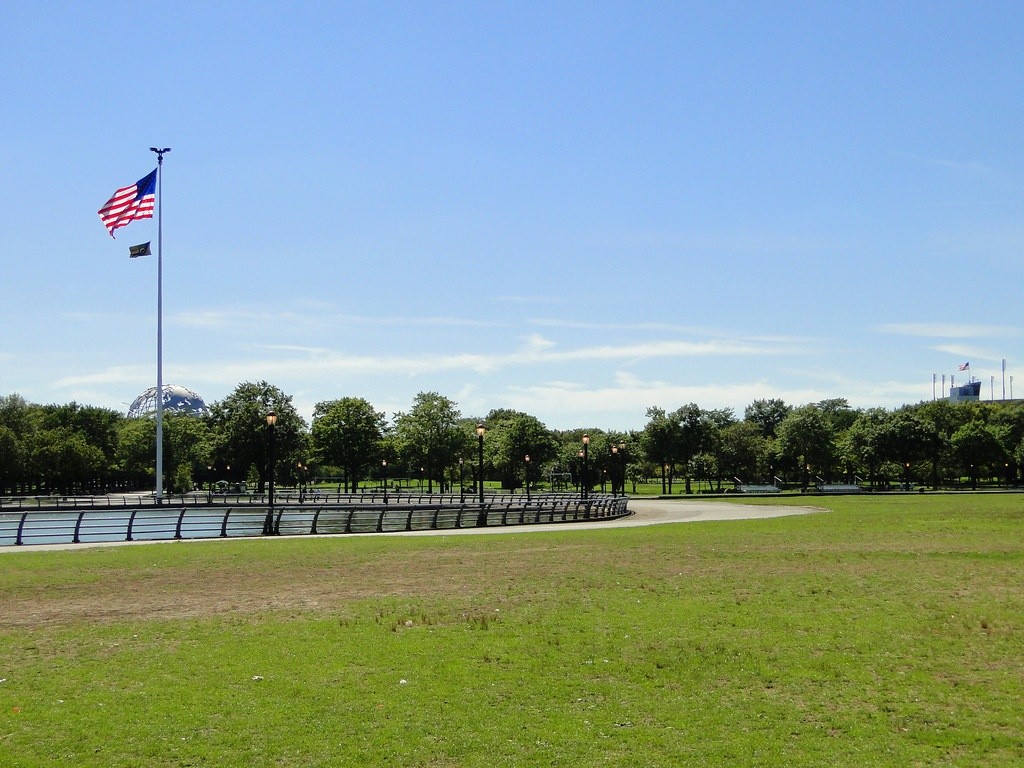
[395,485,401,495]
[910,482,914,491]
[900,482,905,491]
[309,488,314,500]
[316,488,320,501]
[463,487,474,494]
[301,487,307,501]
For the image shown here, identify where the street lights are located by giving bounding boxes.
[226,464,231,490]
[611,445,617,503]
[264,410,277,534]
[420,467,424,493]
[208,466,216,491]
[459,457,465,502]
[524,454,530,504]
[579,450,584,499]
[298,460,308,505]
[476,423,485,507]
[382,459,387,504]
[604,469,606,494]
[582,434,590,503]
[619,440,626,497]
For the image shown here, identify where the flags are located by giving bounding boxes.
[98,167,158,239]
[129,241,151,258]
[959,362,969,371]
